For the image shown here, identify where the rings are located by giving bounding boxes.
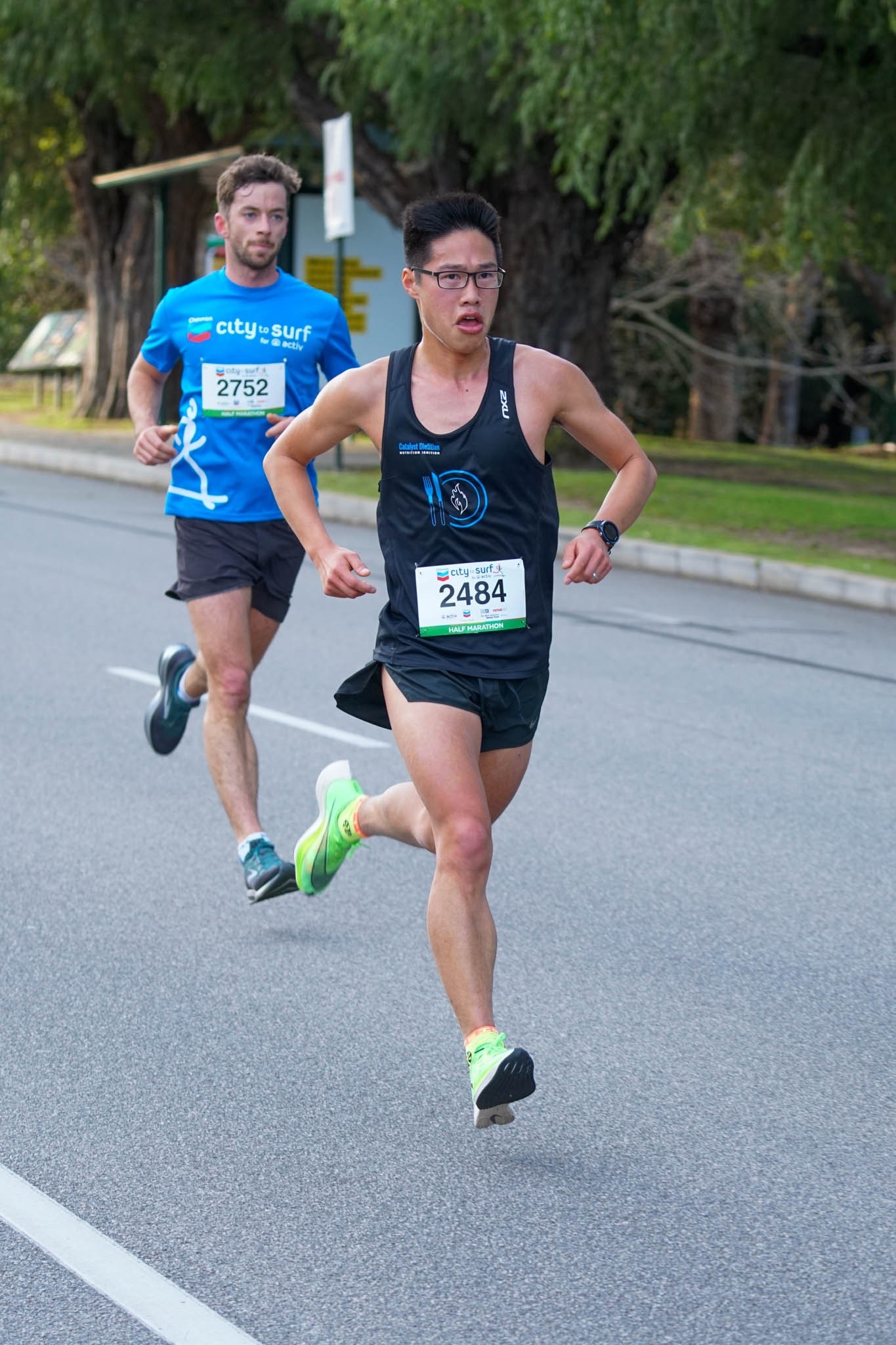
[593,572,600,582]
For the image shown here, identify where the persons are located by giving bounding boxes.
[124,151,360,905]
[259,194,657,1131]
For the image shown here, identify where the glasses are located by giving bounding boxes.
[410,266,506,289]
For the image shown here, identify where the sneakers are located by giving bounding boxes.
[472,1031,536,1129]
[294,758,365,898]
[144,642,202,754]
[244,840,299,905]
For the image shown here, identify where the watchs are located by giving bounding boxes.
[581,520,621,555]
